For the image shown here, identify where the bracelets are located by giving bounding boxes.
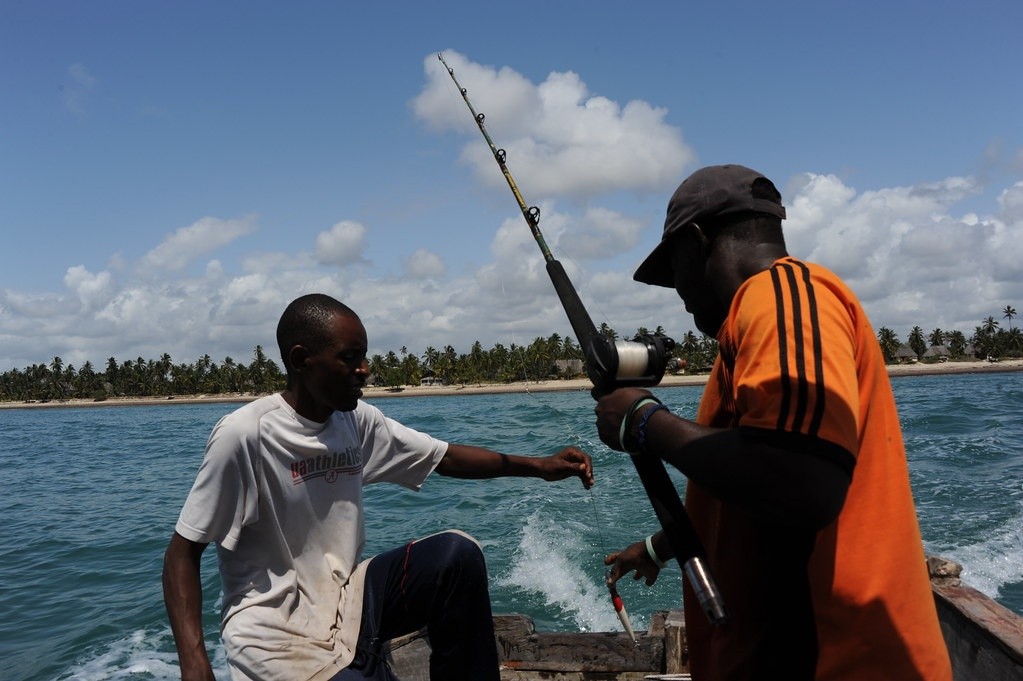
[645,535,667,569]
[619,396,671,456]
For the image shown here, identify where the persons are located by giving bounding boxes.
[161,293,594,681]
[595,165,953,681]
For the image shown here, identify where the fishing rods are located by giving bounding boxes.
[436,49,726,630]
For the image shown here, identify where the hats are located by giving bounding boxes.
[633,165,785,287]
